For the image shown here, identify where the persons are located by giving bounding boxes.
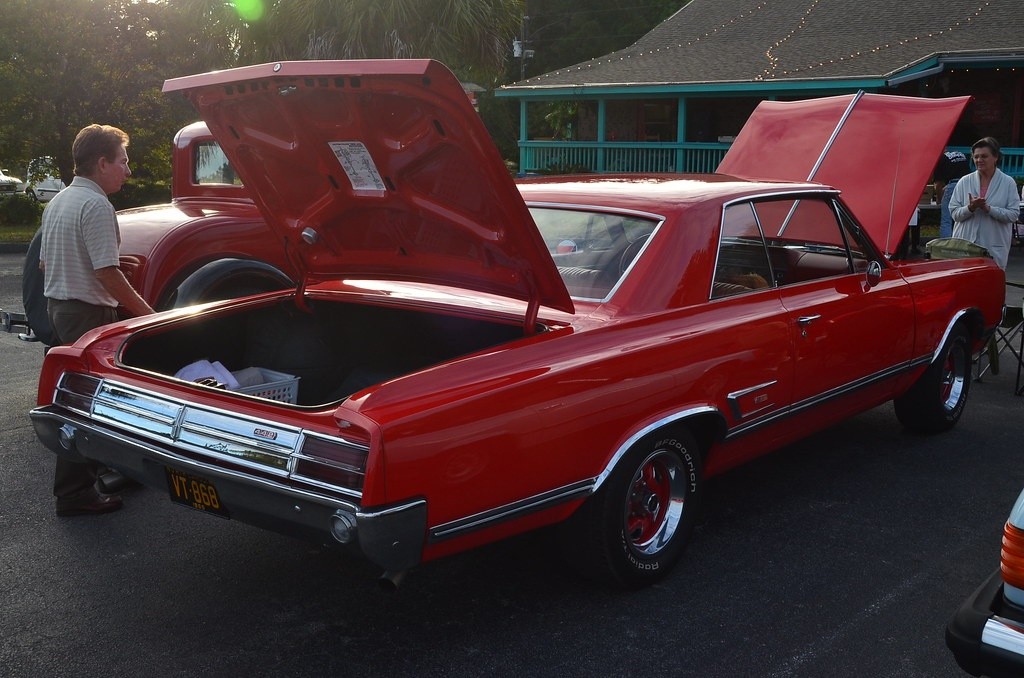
[948,137,1020,271]
[38,124,156,517]
[933,149,971,237]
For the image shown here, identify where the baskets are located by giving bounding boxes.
[232,367,301,404]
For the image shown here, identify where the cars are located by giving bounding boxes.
[26,45,1008,594]
[117,120,299,321]
[0,167,27,199]
[24,155,66,204]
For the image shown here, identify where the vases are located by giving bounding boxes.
[935,180,944,205]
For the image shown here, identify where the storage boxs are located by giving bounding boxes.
[918,191,932,204]
[924,184,934,192]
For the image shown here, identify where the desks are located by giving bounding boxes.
[911,205,942,253]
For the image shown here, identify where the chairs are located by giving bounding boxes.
[554,267,613,288]
[711,282,749,299]
[923,235,1024,395]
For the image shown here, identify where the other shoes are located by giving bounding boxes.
[65,495,124,515]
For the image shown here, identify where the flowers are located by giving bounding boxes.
[933,149,967,182]
